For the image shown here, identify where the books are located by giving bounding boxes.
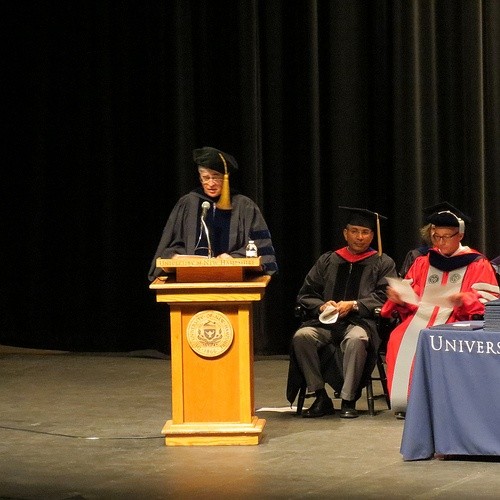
[483,300,500,333]
[430,322,482,331]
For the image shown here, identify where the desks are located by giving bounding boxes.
[400,321,500,461]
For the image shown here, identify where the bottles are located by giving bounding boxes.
[245,240,258,258]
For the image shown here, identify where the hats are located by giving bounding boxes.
[337,206,388,257]
[192,147,249,210]
[419,201,472,241]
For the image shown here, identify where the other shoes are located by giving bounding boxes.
[395,412,406,419]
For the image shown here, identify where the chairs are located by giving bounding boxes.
[293,305,391,417]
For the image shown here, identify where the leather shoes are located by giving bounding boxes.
[302,396,335,417]
[339,398,359,417]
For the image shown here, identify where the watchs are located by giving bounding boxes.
[352,300,359,312]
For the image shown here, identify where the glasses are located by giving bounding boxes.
[347,228,372,236]
[200,176,224,185]
[433,232,459,242]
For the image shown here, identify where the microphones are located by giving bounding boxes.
[200,200,210,231]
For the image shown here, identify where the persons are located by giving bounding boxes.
[382,211,500,419]
[286,206,399,417]
[147,146,279,282]
[400,201,471,279]
